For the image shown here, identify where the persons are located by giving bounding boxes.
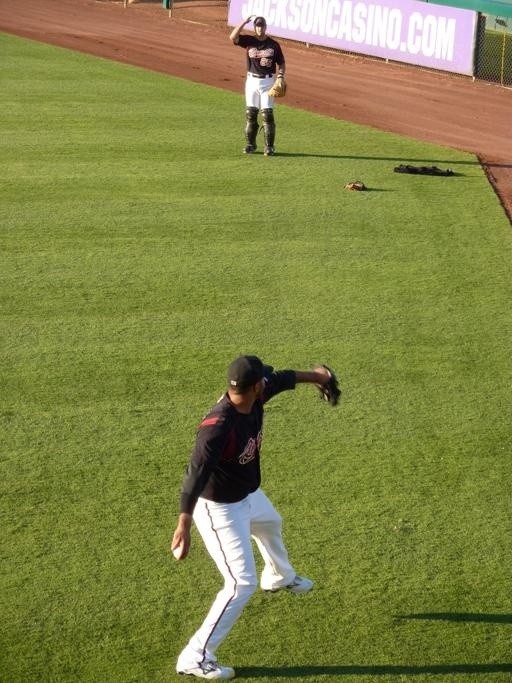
[229,13,286,156]
[169,353,341,679]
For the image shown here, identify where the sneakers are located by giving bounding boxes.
[261,574,313,593]
[243,145,257,153]
[176,661,235,679]
[264,146,274,156]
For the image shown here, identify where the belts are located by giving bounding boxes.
[248,73,273,78]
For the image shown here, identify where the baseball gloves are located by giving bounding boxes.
[268,76,286,98]
[314,364,340,406]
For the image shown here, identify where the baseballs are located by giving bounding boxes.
[173,545,184,560]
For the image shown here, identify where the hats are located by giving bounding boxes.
[228,355,275,387]
[253,17,266,27]
